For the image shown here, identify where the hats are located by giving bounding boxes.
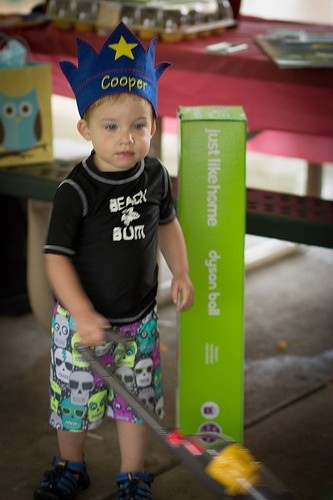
[59,21,172,123]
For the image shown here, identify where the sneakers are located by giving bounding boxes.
[114,472,154,500]
[34,456,89,500]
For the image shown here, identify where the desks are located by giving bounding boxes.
[0,16,333,200]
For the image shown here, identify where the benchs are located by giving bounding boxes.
[0,159,333,319]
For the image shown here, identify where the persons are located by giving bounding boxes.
[31,21,194,500]
